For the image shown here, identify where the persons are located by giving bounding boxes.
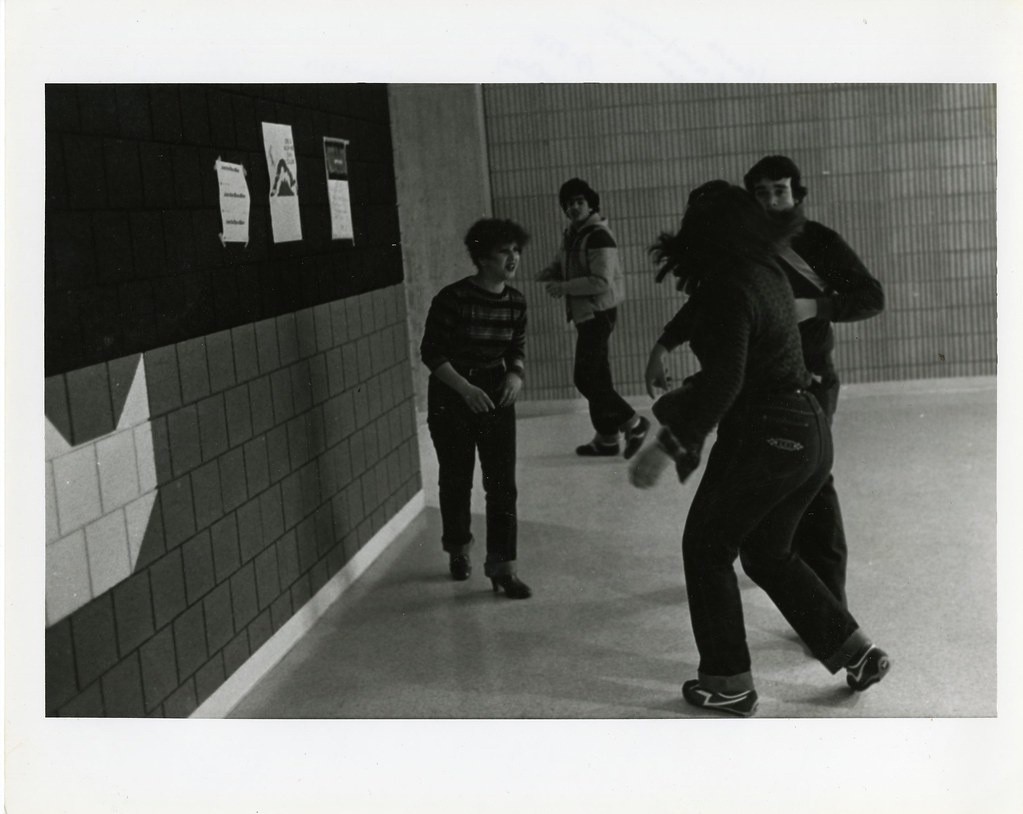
[644,153,886,654]
[416,216,535,601]
[533,177,653,461]
[629,177,893,718]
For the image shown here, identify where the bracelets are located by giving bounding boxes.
[510,364,527,380]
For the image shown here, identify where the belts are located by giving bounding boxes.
[744,387,809,396]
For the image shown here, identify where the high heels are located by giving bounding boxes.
[443,538,476,580]
[483,561,532,599]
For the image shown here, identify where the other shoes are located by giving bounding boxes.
[623,416,650,459]
[576,437,619,457]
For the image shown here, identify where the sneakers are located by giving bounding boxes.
[682,679,759,716]
[847,644,890,692]
